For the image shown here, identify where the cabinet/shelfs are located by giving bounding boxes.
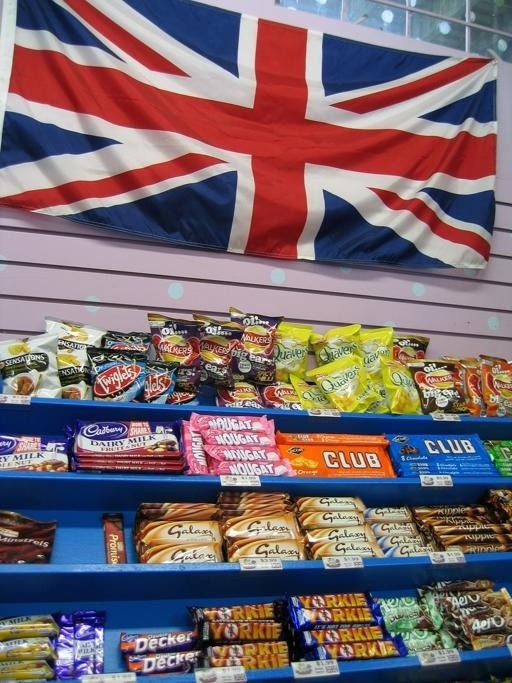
[0,394,512,683]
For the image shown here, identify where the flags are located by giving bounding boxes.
[1,2,498,269]
